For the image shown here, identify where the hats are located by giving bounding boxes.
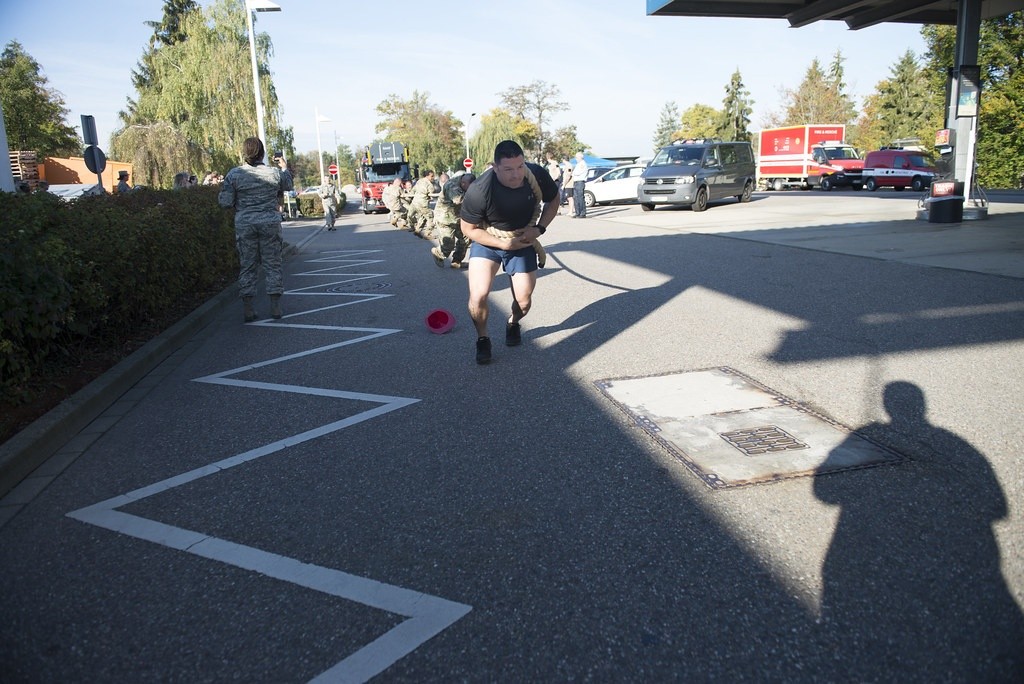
[561,156,569,160]
[119,171,130,176]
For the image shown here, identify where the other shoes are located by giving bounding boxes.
[414,227,423,239]
[392,220,398,228]
[331,224,337,231]
[408,225,415,232]
[573,214,586,218]
[328,224,332,231]
[423,234,436,240]
[431,247,444,267]
[450,262,468,269]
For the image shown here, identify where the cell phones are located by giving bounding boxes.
[274,151,282,163]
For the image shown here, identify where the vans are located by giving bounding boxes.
[637,137,756,212]
[862,145,940,191]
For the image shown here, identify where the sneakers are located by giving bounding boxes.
[505,315,522,346]
[477,336,492,365]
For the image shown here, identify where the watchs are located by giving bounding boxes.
[535,225,546,234]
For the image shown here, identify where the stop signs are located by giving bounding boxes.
[464,158,473,168]
[329,165,338,175]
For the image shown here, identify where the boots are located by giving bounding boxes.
[270,295,282,319]
[243,296,258,321]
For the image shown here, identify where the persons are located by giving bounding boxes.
[117,171,131,191]
[218,138,294,321]
[20,183,30,193]
[290,189,299,198]
[546,152,562,187]
[203,171,224,185]
[572,152,588,218]
[459,140,560,364]
[318,174,339,231]
[382,170,450,241]
[431,173,476,270]
[174,172,198,187]
[562,156,574,216]
[39,182,49,193]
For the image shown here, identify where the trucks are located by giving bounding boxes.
[756,123,865,191]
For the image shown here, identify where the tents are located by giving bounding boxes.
[559,154,617,167]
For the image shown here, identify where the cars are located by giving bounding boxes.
[282,184,321,198]
[545,165,647,208]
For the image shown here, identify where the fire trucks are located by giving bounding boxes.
[354,140,420,214]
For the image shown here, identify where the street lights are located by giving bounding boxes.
[465,113,476,175]
[334,130,342,195]
[245,0,282,168]
[316,107,331,186]
